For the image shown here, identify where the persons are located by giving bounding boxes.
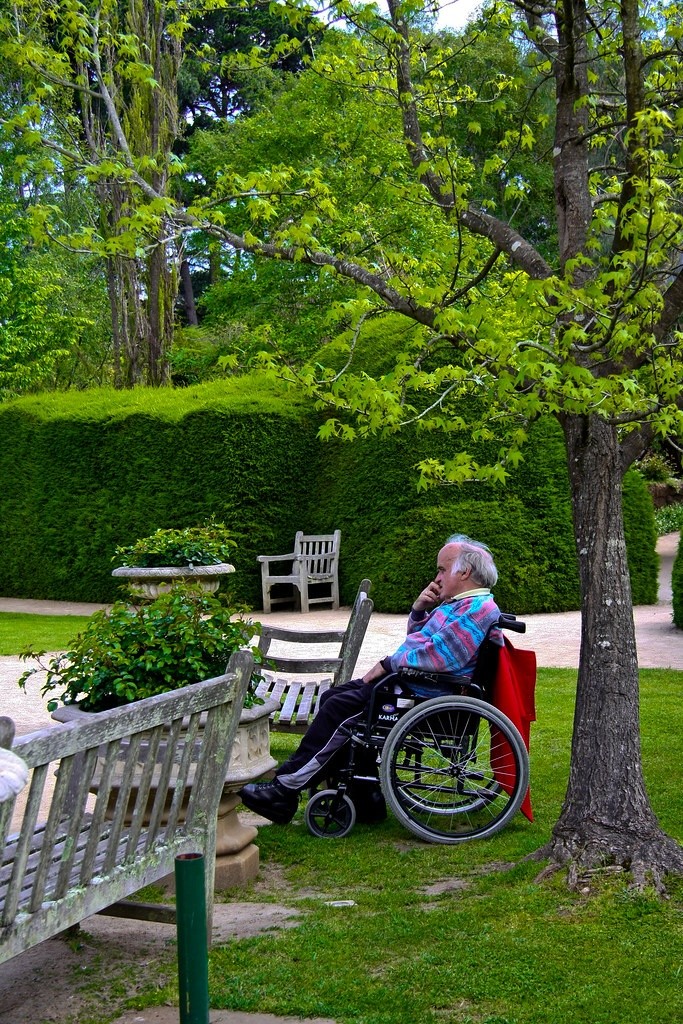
[237,533,504,827]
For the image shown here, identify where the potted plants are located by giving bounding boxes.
[110,508,242,606]
[18,576,282,889]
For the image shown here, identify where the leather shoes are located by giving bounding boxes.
[236,777,298,825]
[326,790,387,823]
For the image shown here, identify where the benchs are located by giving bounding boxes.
[251,578,375,788]
[0,650,255,1024]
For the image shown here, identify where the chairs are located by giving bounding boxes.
[258,530,341,613]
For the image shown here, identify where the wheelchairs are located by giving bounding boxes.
[306,612,531,844]
